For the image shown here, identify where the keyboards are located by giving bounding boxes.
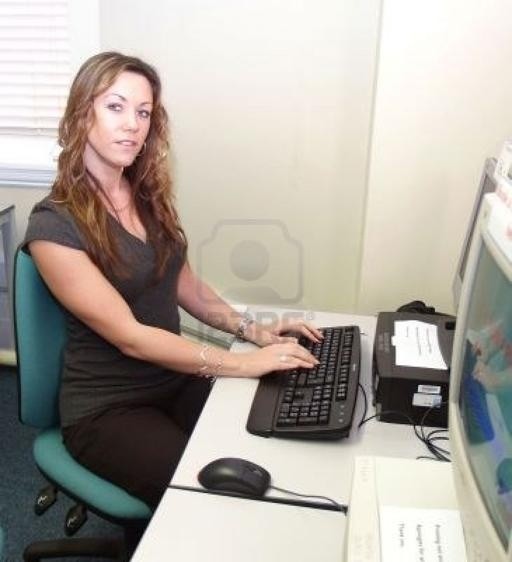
[246,325,360,438]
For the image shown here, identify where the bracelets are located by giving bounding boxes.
[235,316,256,340]
[197,339,225,384]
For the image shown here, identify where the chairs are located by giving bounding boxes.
[10,242,154,561]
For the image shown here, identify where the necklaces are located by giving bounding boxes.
[108,195,131,214]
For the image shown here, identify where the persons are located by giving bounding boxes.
[20,48,328,516]
[470,313,511,429]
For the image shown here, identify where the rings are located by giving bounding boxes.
[279,356,287,363]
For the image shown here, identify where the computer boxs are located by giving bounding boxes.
[372,312,456,427]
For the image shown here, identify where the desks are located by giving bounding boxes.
[134,307,466,562]
[1,201,21,326]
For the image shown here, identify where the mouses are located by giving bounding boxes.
[198,459,270,497]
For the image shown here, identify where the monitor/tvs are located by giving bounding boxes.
[452,157,498,311]
[447,191,512,562]
